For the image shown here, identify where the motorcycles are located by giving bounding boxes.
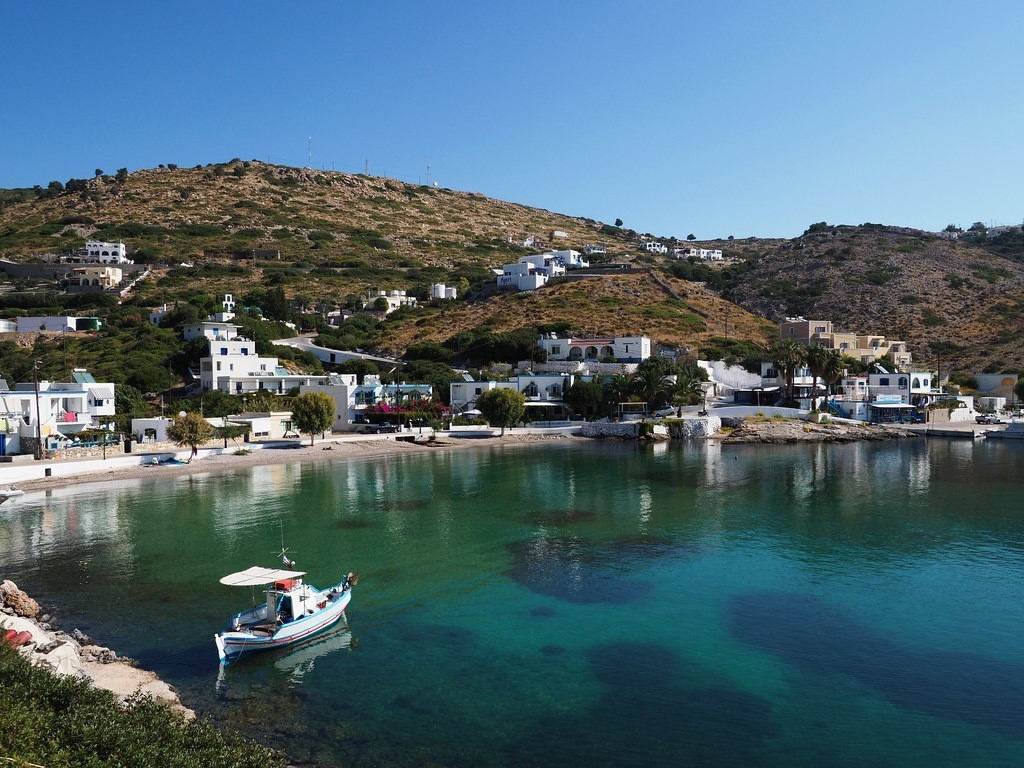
[698,409,709,417]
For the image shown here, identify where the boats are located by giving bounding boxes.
[980,417,1024,439]
[214,519,358,669]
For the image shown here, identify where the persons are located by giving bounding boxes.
[912,394,921,406]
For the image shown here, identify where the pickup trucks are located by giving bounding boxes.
[370,421,399,434]
[975,413,1000,425]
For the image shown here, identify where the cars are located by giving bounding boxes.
[651,405,676,417]
[1012,408,1024,416]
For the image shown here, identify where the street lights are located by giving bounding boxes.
[34,359,43,461]
